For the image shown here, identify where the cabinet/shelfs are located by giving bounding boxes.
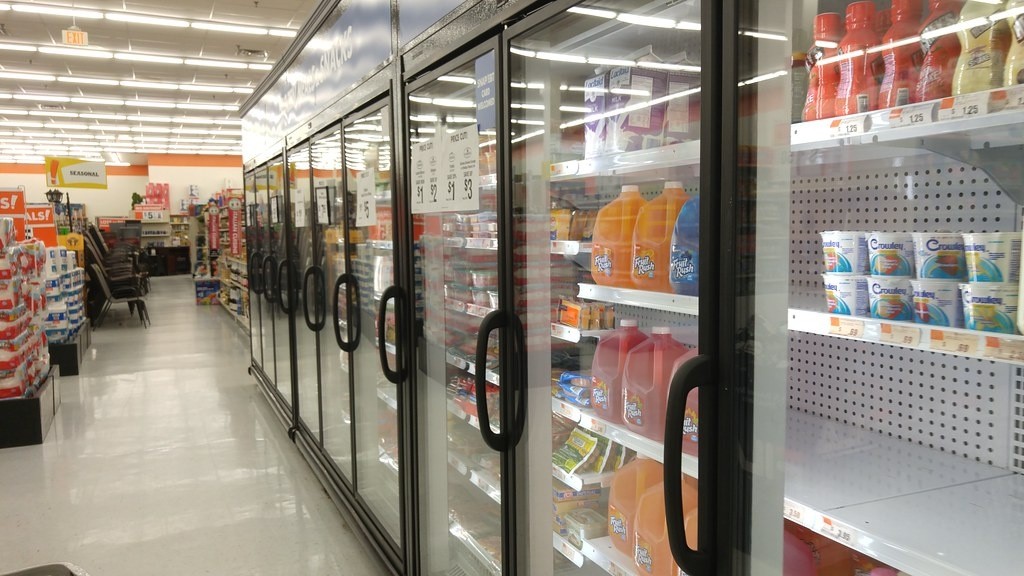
[83,222,151,329]
[140,204,250,338]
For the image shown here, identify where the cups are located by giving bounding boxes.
[818,230,1024,336]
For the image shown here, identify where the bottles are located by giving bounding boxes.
[588,181,698,576]
[799,0,1024,123]
